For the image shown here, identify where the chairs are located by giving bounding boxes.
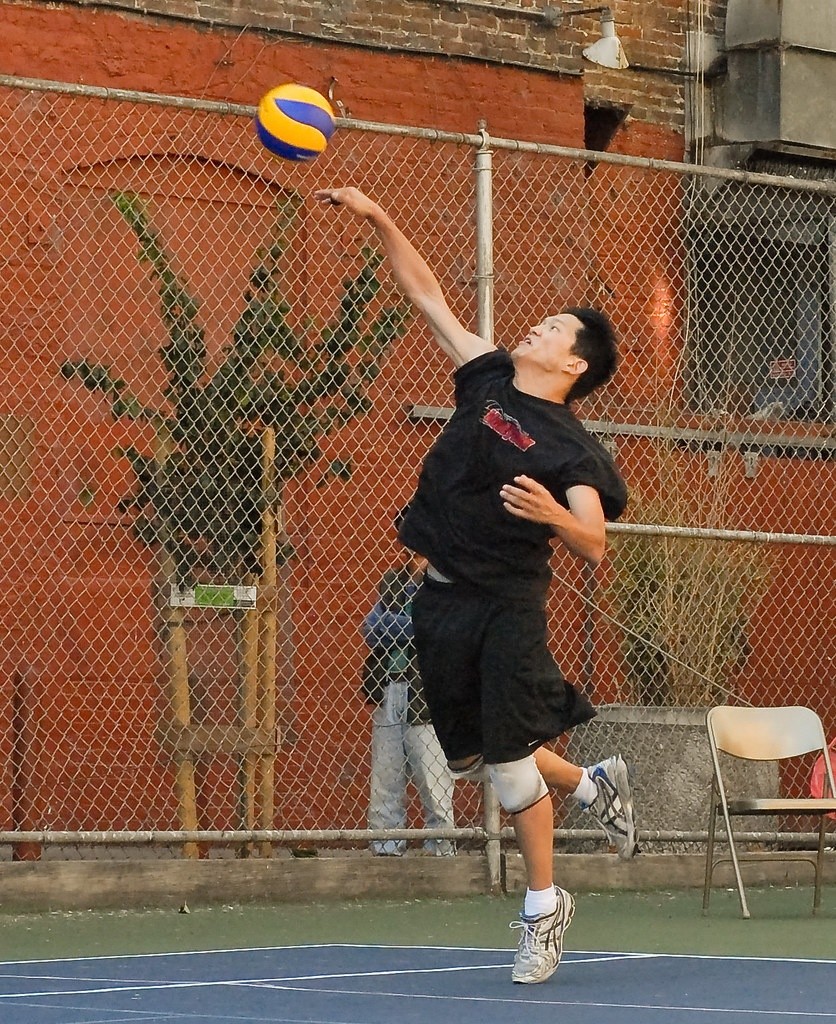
[701,706,836,920]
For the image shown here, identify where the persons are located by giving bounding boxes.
[359,504,458,856]
[314,187,638,981]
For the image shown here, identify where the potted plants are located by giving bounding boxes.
[563,387,778,855]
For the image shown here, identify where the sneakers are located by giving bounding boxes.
[578,753,641,861]
[509,885,576,984]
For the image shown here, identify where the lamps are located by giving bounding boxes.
[557,6,629,69]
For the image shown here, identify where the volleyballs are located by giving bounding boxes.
[253,81,339,164]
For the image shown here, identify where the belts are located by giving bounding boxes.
[385,672,407,682]
